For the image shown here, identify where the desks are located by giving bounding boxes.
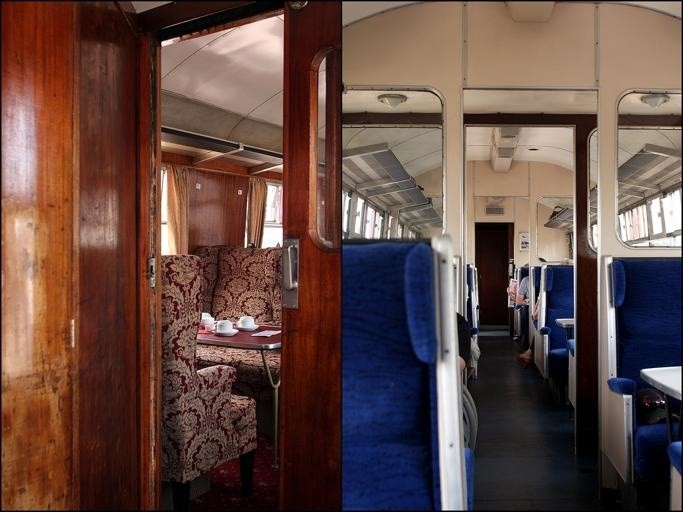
[193,323,285,470]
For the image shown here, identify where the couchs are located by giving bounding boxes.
[198,244,281,451]
[160,255,258,509]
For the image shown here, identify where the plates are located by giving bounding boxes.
[237,324,258,332]
[210,329,237,336]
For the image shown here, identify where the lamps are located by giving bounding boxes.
[378,93,407,111]
[638,93,672,110]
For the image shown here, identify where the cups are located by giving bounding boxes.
[236,316,254,328]
[201,316,214,328]
[216,320,232,333]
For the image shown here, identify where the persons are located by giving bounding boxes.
[506,257,550,310]
[456,309,473,376]
[517,291,540,365]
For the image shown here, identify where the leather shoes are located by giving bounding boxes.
[516,352,530,365]
